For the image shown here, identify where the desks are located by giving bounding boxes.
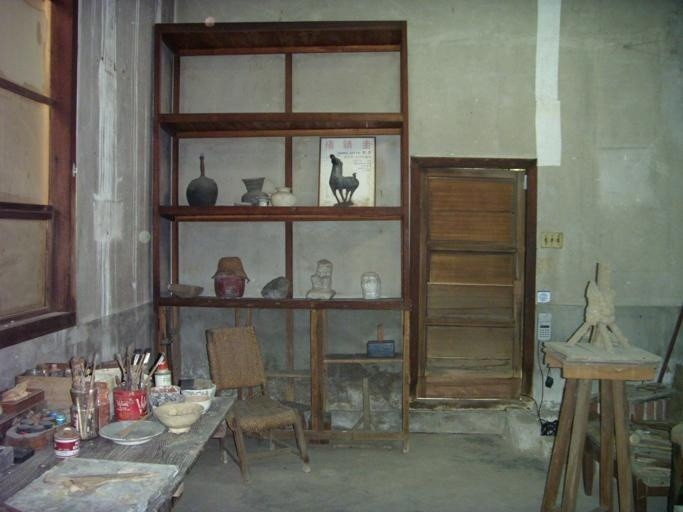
[539,337,664,512]
[0,387,240,511]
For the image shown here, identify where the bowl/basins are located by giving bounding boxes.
[153,404,201,435]
[186,396,211,415]
[181,384,216,400]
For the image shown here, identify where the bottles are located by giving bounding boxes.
[153,362,172,389]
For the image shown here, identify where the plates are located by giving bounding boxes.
[98,420,165,445]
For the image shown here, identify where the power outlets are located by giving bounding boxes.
[539,230,565,251]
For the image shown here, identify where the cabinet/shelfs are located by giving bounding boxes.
[150,20,412,455]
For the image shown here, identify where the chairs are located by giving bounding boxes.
[204,325,312,484]
[577,300,682,512]
